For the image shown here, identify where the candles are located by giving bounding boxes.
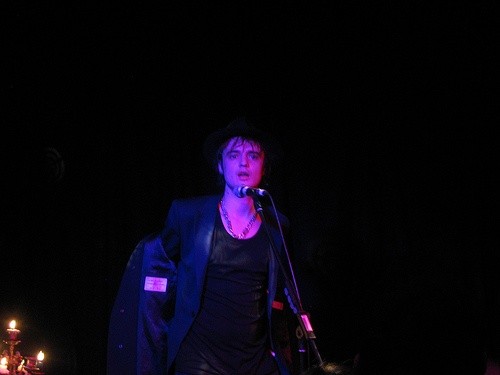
[36,350,45,364]
[7,320,20,341]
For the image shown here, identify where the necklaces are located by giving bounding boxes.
[220,202,257,239]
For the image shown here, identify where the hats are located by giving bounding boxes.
[203,120,272,164]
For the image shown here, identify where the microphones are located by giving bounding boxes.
[233,184,267,198]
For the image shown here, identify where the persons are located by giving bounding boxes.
[106,125,292,375]
